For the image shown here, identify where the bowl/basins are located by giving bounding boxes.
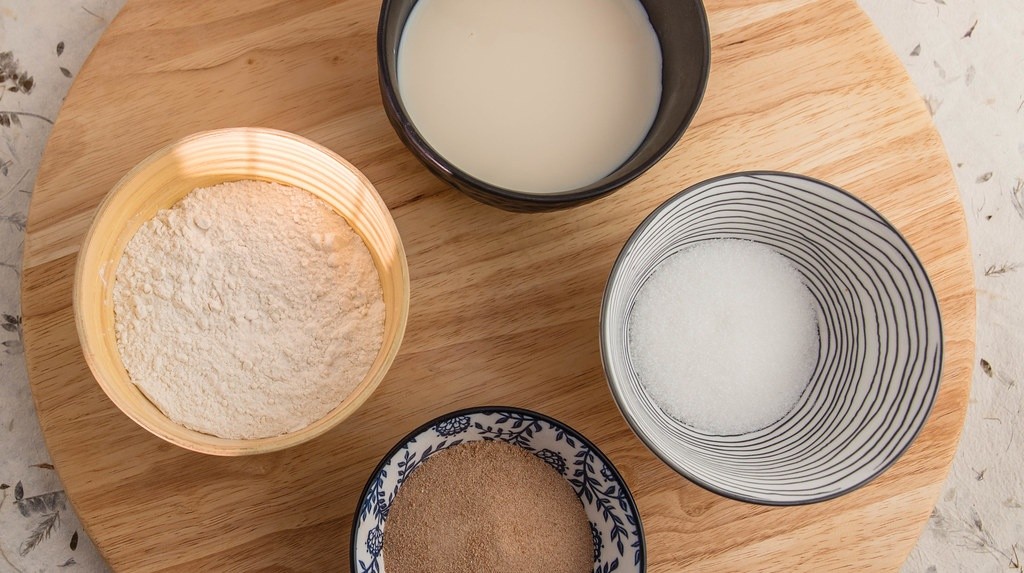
[74,127,411,457]
[350,406,646,573]
[598,170,944,507]
[377,0,711,209]
